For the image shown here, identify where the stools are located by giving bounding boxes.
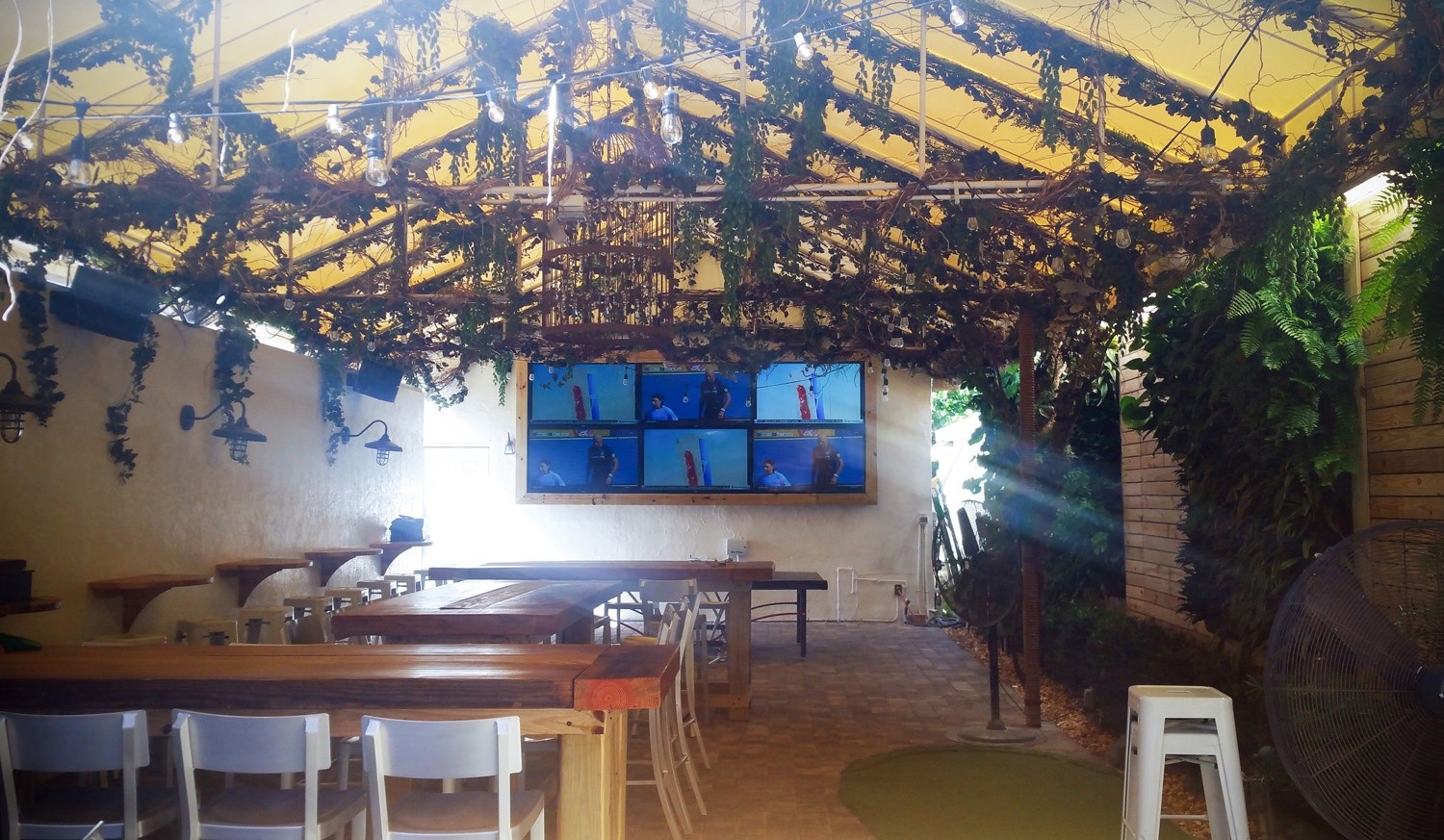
[1121,685,1250,840]
[89,570,446,790]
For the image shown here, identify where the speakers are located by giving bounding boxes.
[58,266,160,340]
[354,359,404,405]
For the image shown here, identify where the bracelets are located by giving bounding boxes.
[608,473,613,477]
[833,473,838,477]
[720,407,725,412]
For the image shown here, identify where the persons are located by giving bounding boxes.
[809,435,843,486]
[644,393,679,421]
[585,432,618,485]
[753,460,791,487]
[532,459,566,488]
[698,367,731,420]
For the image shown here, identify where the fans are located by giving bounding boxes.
[931,500,1036,743]
[1264,521,1444,840]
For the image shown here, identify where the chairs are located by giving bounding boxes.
[360,715,545,840]
[0,709,200,840]
[594,555,733,840]
[171,709,368,840]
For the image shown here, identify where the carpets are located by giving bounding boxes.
[839,743,1191,840]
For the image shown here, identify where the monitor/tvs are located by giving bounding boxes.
[526,360,866,495]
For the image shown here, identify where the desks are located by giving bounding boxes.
[752,572,828,658]
[217,559,313,608]
[89,574,215,634]
[333,581,622,644]
[306,547,383,587]
[0,595,64,616]
[0,645,680,840]
[429,561,776,721]
[370,541,432,576]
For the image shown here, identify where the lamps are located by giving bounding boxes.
[181,398,267,461]
[0,353,46,444]
[342,420,402,465]
